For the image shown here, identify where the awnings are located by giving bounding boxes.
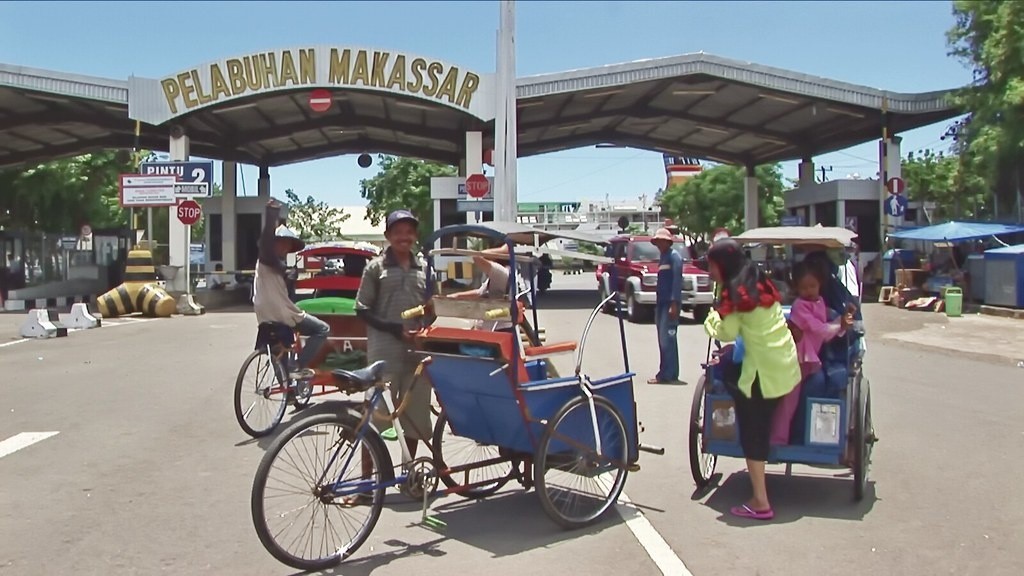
[885,221,1024,297]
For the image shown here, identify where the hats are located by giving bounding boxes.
[258,224,305,253]
[386,209,419,230]
[650,228,673,247]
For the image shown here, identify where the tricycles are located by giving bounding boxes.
[234,238,387,439]
[689,223,879,499]
[251,217,665,569]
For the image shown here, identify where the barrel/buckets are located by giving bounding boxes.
[944,287,963,317]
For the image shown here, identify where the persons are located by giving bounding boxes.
[704,238,863,520]
[342,210,434,506]
[252,199,331,405]
[647,227,683,384]
[207,263,230,290]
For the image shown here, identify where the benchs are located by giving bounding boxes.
[295,296,357,314]
[733,305,847,391]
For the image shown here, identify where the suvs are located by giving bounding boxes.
[596,234,715,323]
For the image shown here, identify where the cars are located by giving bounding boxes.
[190,244,205,271]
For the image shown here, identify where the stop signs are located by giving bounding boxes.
[177,200,201,224]
[466,173,489,198]
[308,88,331,112]
[887,177,904,195]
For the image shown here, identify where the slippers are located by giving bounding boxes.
[338,490,375,509]
[400,484,429,497]
[730,504,774,519]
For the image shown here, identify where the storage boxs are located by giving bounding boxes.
[890,268,931,310]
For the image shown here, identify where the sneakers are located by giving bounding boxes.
[287,395,296,406]
[288,367,315,379]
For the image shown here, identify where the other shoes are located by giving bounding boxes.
[647,373,679,384]
